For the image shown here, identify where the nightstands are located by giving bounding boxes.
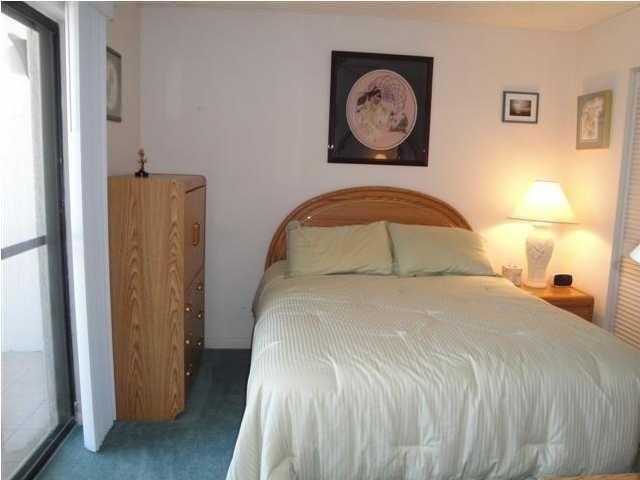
[520,281,594,323]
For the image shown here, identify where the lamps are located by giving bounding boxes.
[505,173,577,289]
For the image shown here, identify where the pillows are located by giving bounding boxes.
[384,221,499,279]
[282,217,396,280]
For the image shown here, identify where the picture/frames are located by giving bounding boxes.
[106,45,124,125]
[500,89,542,125]
[574,89,613,154]
[325,49,434,168]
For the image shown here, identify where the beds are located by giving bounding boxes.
[228,182,639,480]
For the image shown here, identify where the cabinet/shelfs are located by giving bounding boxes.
[106,172,211,426]
